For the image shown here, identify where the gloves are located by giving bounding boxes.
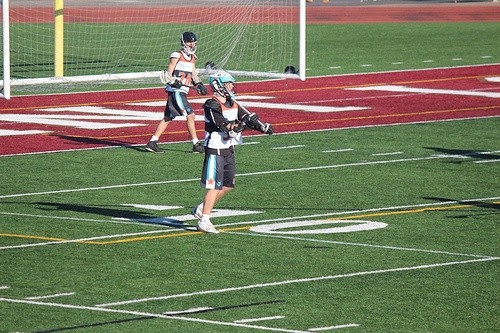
[197,82,208,96]
[172,80,183,89]
[243,112,259,127]
[256,122,273,134]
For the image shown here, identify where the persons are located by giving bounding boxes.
[146,31,207,153]
[194,68,272,233]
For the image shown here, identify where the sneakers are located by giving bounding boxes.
[191,207,203,219]
[198,217,219,233]
[193,143,205,153]
[145,141,165,154]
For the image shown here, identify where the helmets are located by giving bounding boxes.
[181,32,198,54]
[209,70,237,97]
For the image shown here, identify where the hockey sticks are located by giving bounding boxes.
[212,79,267,128]
[159,71,201,91]
